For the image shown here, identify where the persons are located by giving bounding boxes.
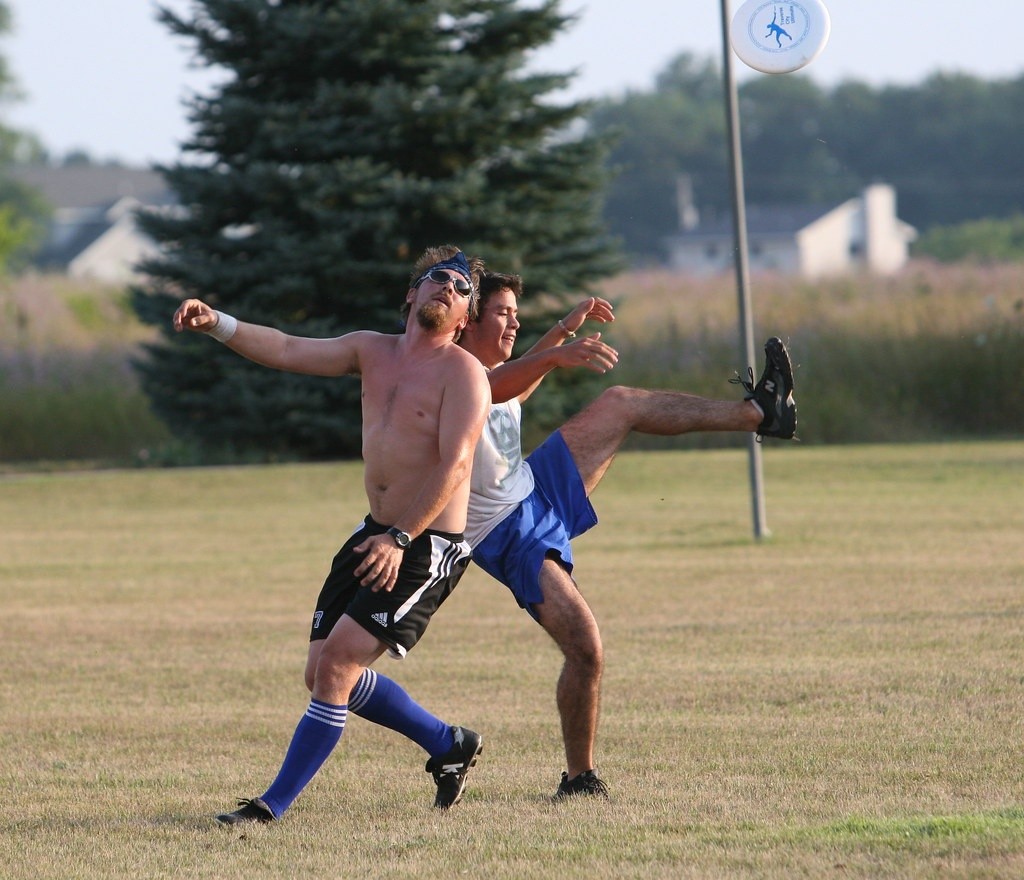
[457,271,797,797]
[173,245,491,826]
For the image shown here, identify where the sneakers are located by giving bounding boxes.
[425,726,484,811]
[728,336,799,443]
[216,796,278,828]
[553,768,610,800]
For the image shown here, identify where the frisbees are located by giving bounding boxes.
[728,0,833,75]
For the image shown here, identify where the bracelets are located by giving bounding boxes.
[203,308,238,344]
[558,319,576,339]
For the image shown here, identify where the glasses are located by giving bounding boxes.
[413,269,474,316]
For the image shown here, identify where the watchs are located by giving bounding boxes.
[386,526,412,551]
